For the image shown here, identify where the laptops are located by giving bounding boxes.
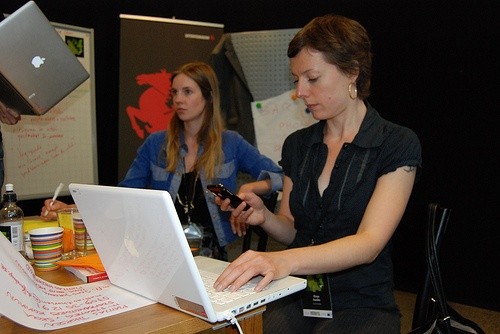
[0,0,90,116]
[69,183,307,323]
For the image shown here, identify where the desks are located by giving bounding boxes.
[0,216,266,334]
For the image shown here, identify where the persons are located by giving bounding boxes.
[0,12,22,206]
[215,15,423,334]
[40,61,283,262]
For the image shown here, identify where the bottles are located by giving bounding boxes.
[0,183,25,259]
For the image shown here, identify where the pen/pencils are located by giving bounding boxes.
[43,183,64,216]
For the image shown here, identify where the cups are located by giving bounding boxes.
[56,209,87,260]
[28,227,64,271]
[72,207,96,256]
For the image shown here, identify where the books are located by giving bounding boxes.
[51,252,108,283]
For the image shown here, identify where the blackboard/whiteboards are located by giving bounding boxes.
[0,13,98,206]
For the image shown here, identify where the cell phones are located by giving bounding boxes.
[207,184,250,211]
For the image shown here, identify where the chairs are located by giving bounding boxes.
[384,203,450,334]
